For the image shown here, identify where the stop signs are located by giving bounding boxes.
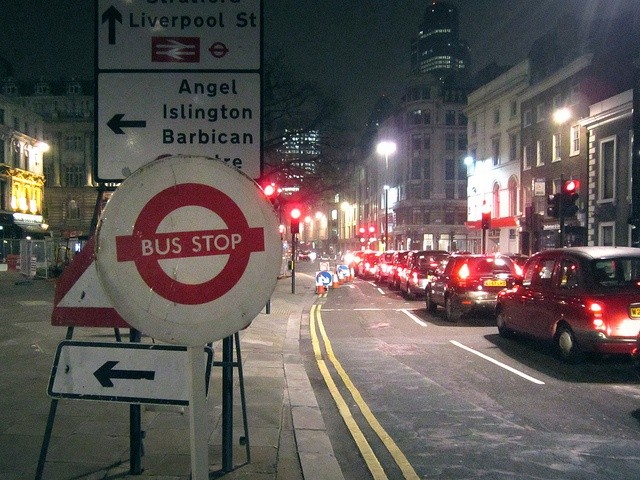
[90,153,282,346]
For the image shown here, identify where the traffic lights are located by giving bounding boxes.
[547,192,559,218]
[503,252,529,269]
[367,226,375,246]
[358,226,366,245]
[559,173,580,218]
[481,204,491,229]
[256,176,276,204]
[290,208,301,233]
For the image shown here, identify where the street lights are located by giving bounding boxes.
[376,139,398,250]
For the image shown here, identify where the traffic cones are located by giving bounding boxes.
[350,268,355,281]
[332,269,339,288]
[338,269,345,285]
[345,268,351,282]
[315,273,326,294]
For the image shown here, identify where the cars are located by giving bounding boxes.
[388,250,409,287]
[348,251,364,273]
[375,251,396,284]
[284,247,316,263]
[400,250,450,298]
[356,252,376,279]
[424,254,524,321]
[494,244,640,363]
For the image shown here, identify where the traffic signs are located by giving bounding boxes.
[95,70,265,182]
[94,0,264,70]
